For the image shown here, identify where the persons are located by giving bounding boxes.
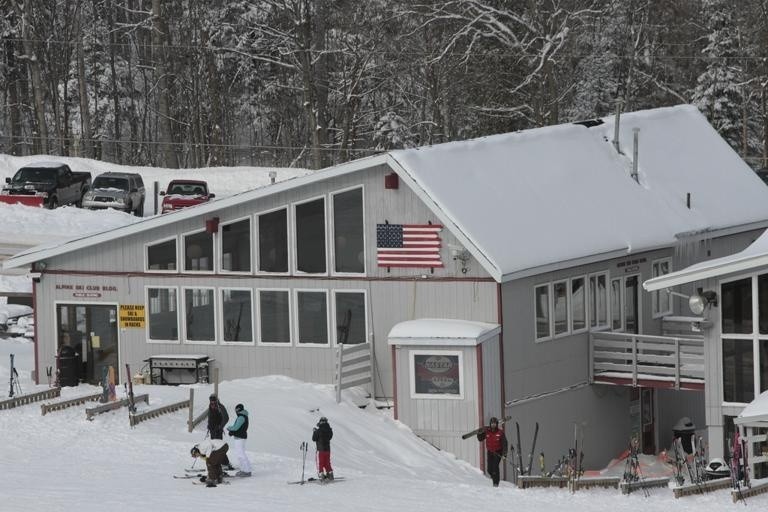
[478,416,510,489]
[312,416,334,482]
[193,394,228,439]
[191,437,229,489]
[226,403,253,477]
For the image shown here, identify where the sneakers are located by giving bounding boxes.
[236,471,251,476]
[206,478,221,485]
[318,471,333,479]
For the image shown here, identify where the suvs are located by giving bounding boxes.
[156,180,216,216]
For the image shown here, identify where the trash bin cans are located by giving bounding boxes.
[672,417,696,453]
[57,346,78,386]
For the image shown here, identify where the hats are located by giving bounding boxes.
[490,417,498,427]
[236,404,243,410]
[210,395,217,401]
[191,448,198,457]
[319,417,327,424]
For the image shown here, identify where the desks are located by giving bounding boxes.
[143,354,215,386]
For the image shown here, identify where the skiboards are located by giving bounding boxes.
[574,422,584,482]
[173,468,235,479]
[672,434,711,493]
[462,416,512,439]
[288,476,346,484]
[515,421,539,489]
[623,437,651,498]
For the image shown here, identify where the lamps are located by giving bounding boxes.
[206,217,219,230]
[384,172,399,189]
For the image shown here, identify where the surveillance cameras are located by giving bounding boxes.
[450,249,459,260]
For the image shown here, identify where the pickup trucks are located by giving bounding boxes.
[3,156,93,209]
[86,168,147,217]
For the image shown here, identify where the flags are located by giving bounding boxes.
[374,223,442,270]
